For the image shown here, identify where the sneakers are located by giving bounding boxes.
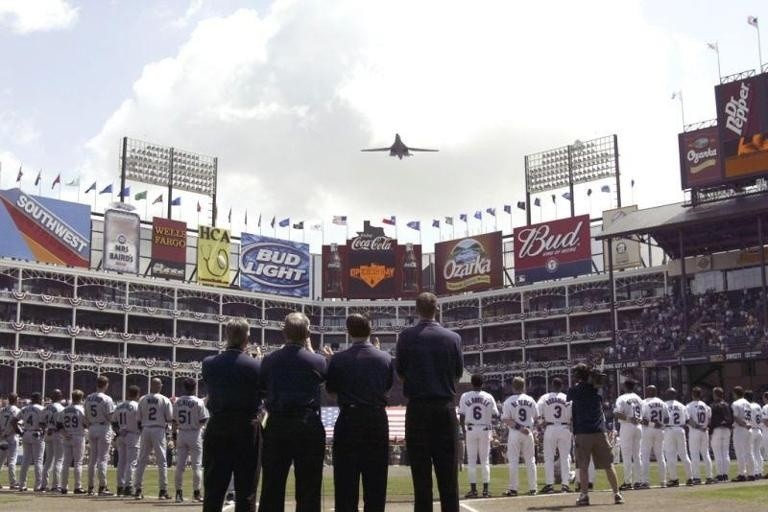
[576,494,589,505]
[40,487,204,502]
[464,485,570,498]
[615,493,624,504]
[226,493,236,504]
[619,473,768,490]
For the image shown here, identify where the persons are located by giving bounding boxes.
[1,282,261,362]
[259,312,327,511]
[459,373,768,497]
[434,288,767,363]
[395,292,464,512]
[325,313,394,511]
[202,317,260,511]
[0,376,209,502]
[566,363,625,507]
[407,313,415,326]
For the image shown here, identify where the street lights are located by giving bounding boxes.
[706,39,722,85]
[116,136,217,227]
[523,132,620,227]
[671,88,685,131]
[746,16,764,75]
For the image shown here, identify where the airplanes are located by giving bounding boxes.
[360,133,439,161]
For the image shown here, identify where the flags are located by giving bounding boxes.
[587,189,592,196]
[17,166,182,206]
[534,192,570,207]
[602,185,610,192]
[197,201,303,228]
[748,16,756,25]
[382,216,420,230]
[311,215,348,228]
[433,201,526,228]
[707,41,716,50]
